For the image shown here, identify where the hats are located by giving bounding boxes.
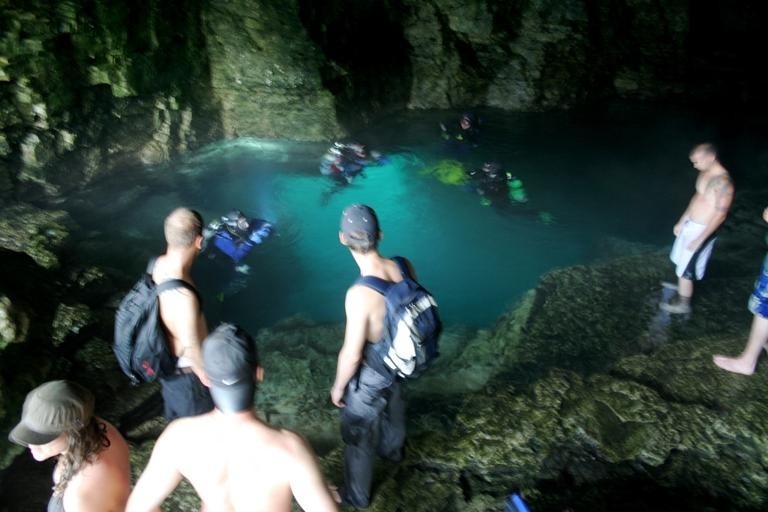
[340,203,379,244]
[10,380,94,445]
[201,320,256,413]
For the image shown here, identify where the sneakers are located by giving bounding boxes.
[659,296,693,314]
[660,277,678,290]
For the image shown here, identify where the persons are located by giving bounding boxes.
[439,113,484,150]
[659,141,735,315]
[200,207,273,265]
[317,141,387,207]
[329,202,417,508]
[125,321,341,512]
[145,205,266,422]
[466,159,558,228]
[713,205,768,376]
[9,378,132,512]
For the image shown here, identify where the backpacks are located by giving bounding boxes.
[355,255,443,386]
[112,257,204,388]
